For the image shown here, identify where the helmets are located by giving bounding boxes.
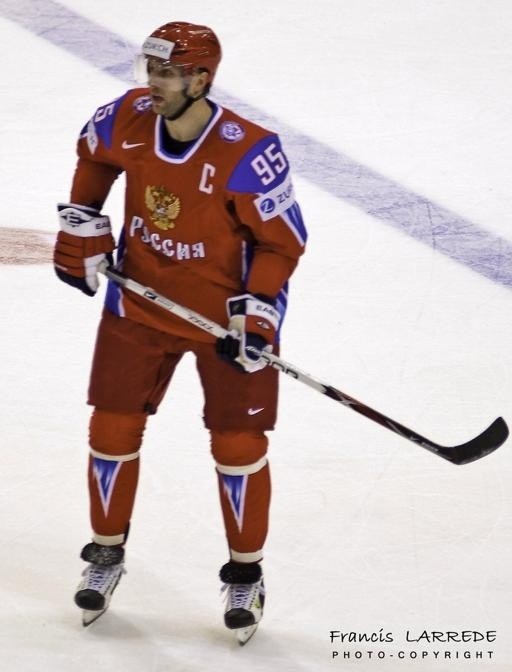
[141,21,222,92]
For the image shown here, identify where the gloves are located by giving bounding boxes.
[215,293,281,373]
[53,202,116,297]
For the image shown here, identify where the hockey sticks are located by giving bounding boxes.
[96,260,509,465]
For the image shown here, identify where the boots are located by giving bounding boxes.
[220,573,266,630]
[74,557,128,611]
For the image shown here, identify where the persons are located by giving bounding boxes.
[52,20,308,630]
